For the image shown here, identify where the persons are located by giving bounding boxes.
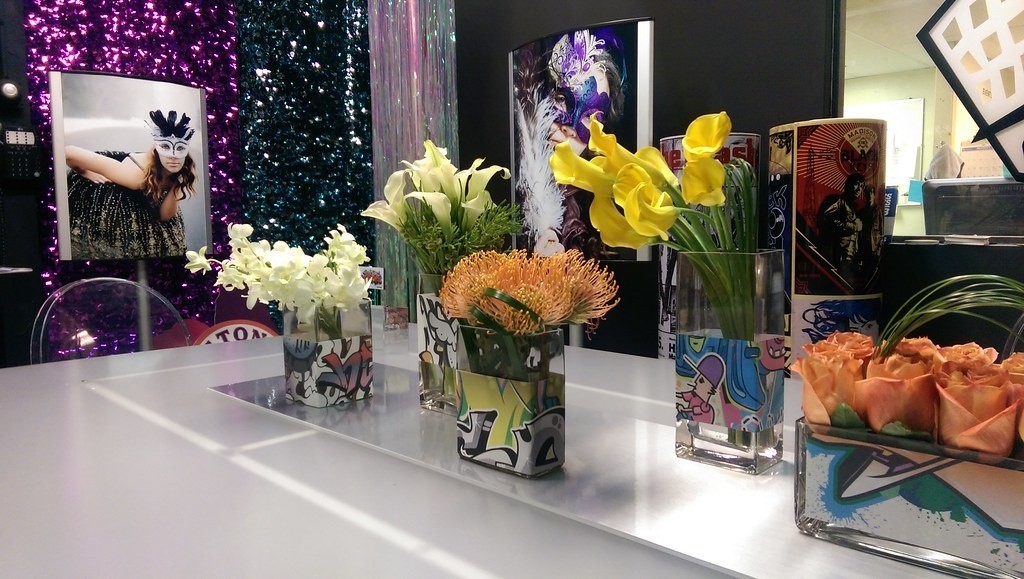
[66,111,200,260]
[513,30,626,260]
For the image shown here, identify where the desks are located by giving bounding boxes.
[0,319,956,579]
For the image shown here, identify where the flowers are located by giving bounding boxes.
[550,110,777,450]
[362,141,529,274]
[438,249,620,335]
[788,274,1024,464]
[182,219,369,338]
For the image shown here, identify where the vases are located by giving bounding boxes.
[455,328,566,480]
[675,247,785,475]
[415,272,462,418]
[794,417,1024,579]
[283,297,374,409]
